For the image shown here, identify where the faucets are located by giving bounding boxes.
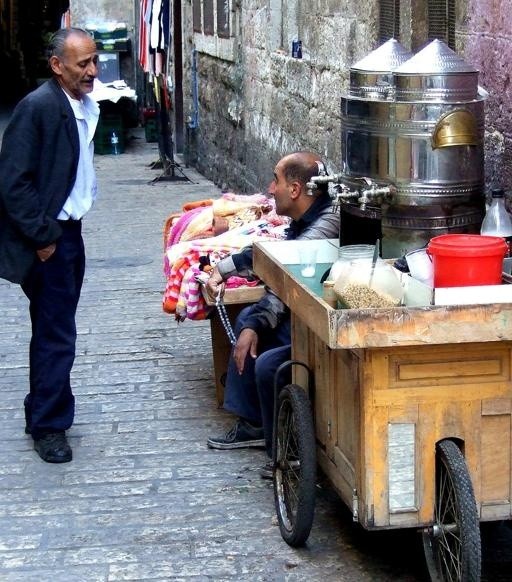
[306,160,340,196]
[331,184,359,215]
[356,177,392,212]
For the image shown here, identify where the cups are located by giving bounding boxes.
[297,247,318,278]
[404,247,433,287]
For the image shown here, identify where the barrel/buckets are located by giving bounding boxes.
[426,234,509,288]
[339,37,416,258]
[382,38,485,254]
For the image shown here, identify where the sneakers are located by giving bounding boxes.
[207,418,266,450]
[34,431,72,463]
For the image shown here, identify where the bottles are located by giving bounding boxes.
[109,131,121,155]
[480,187,512,274]
[325,243,405,310]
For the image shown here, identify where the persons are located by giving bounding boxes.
[205,152,343,449]
[0,27,104,464]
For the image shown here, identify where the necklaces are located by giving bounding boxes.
[215,283,238,346]
